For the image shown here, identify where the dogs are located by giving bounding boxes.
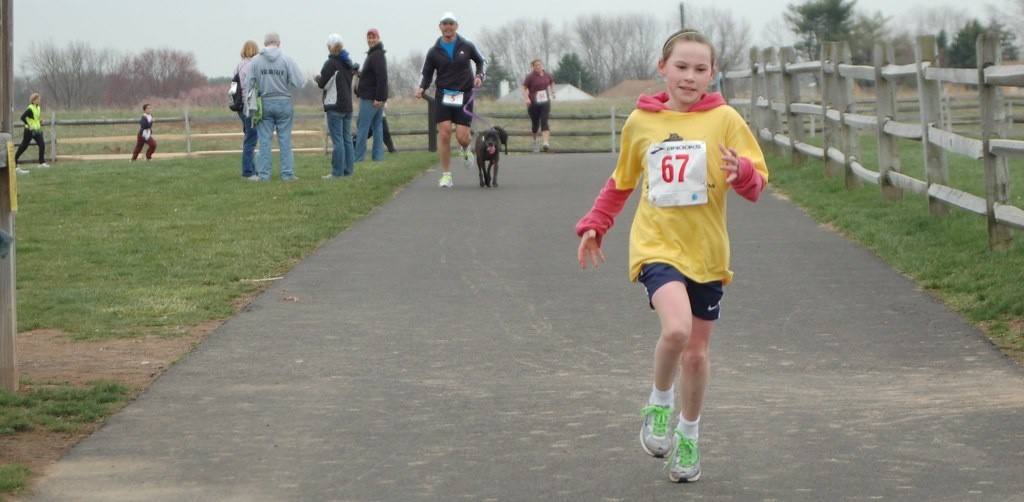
[494,126,508,155]
[476,131,502,188]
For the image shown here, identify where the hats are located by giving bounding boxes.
[366,27,380,36]
[438,11,458,27]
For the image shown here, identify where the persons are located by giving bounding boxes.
[413,12,486,188]
[522,59,556,154]
[575,28,769,483]
[15,92,51,168]
[232,40,259,180]
[132,104,157,161]
[247,33,303,181]
[312,33,356,179]
[354,28,397,162]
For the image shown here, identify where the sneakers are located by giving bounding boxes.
[460,145,474,169]
[438,176,453,187]
[532,139,540,154]
[639,392,674,458]
[663,429,702,482]
[543,142,550,152]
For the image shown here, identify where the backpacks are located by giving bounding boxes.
[228,61,250,109]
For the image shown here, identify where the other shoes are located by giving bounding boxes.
[17,166,24,172]
[38,163,50,168]
[241,174,260,182]
[322,173,335,180]
